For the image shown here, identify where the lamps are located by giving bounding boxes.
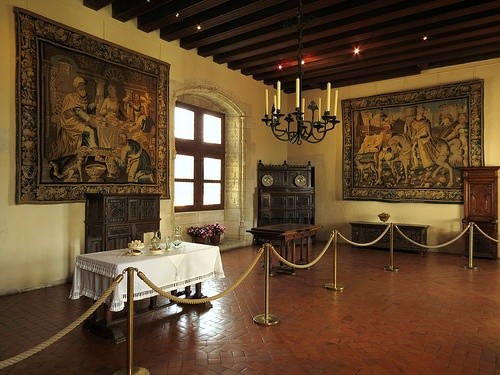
[262,0,341,146]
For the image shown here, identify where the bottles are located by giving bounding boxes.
[151,230,160,249]
[172,226,181,247]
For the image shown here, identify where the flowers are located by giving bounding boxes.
[187,225,209,238]
[206,223,225,237]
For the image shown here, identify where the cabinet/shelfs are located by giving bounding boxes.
[84,191,162,252]
[459,165,500,259]
[255,157,316,247]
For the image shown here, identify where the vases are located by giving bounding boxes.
[210,236,220,246]
[192,236,204,244]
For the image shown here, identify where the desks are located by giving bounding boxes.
[245,222,323,276]
[349,221,430,254]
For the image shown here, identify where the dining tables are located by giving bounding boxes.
[69,240,225,344]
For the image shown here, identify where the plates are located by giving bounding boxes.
[262,175,273,187]
[295,175,307,187]
